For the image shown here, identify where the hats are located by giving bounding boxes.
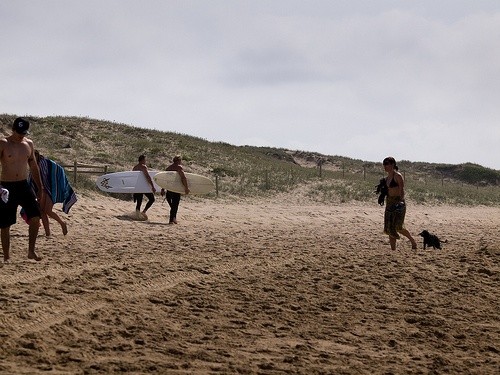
[13,117,30,135]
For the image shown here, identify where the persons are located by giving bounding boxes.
[28,150,78,236]
[161,156,191,224]
[0,117,44,262]
[131,154,156,220]
[377,157,417,250]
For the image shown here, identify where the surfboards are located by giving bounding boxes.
[153,171,215,195]
[96,170,168,193]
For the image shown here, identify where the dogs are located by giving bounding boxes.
[419,228,448,251]
